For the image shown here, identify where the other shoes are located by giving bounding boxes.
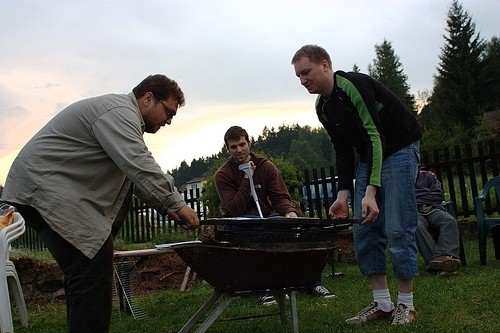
[430,255,461,271]
[436,270,458,276]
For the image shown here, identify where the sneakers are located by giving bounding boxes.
[345,300,398,327]
[312,285,336,299]
[390,303,417,327]
[258,296,278,306]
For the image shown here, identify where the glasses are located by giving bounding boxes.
[152,95,174,119]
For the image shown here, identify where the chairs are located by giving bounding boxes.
[473,176,500,266]
[0,211,27,333]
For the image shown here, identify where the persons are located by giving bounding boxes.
[214,126,336,306]
[291,44,424,325]
[415,171,461,276]
[2,74,201,333]
